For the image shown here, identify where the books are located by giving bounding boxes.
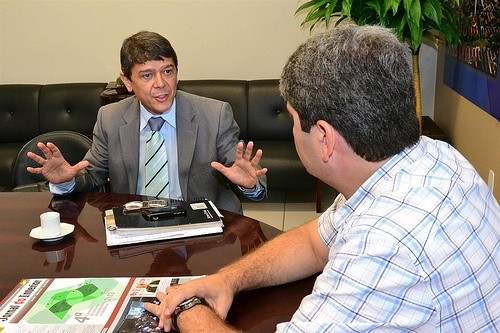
[111,196,224,236]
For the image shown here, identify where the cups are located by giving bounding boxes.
[40,212,61,236]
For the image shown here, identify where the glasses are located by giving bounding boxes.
[122,200,167,212]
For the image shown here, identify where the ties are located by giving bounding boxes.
[144,117,170,199]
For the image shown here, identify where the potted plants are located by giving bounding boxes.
[293,0,469,145]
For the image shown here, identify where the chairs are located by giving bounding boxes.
[10,129,111,194]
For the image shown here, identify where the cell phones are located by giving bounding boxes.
[142,206,186,221]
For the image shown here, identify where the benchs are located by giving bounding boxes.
[0,77,324,214]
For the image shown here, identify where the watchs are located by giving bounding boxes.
[170,297,212,333]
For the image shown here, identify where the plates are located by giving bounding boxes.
[29,223,74,241]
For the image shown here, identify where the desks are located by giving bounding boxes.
[0,191,323,333]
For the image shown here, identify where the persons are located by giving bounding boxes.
[43,192,269,276]
[26,30,269,215]
[145,24,500,333]
